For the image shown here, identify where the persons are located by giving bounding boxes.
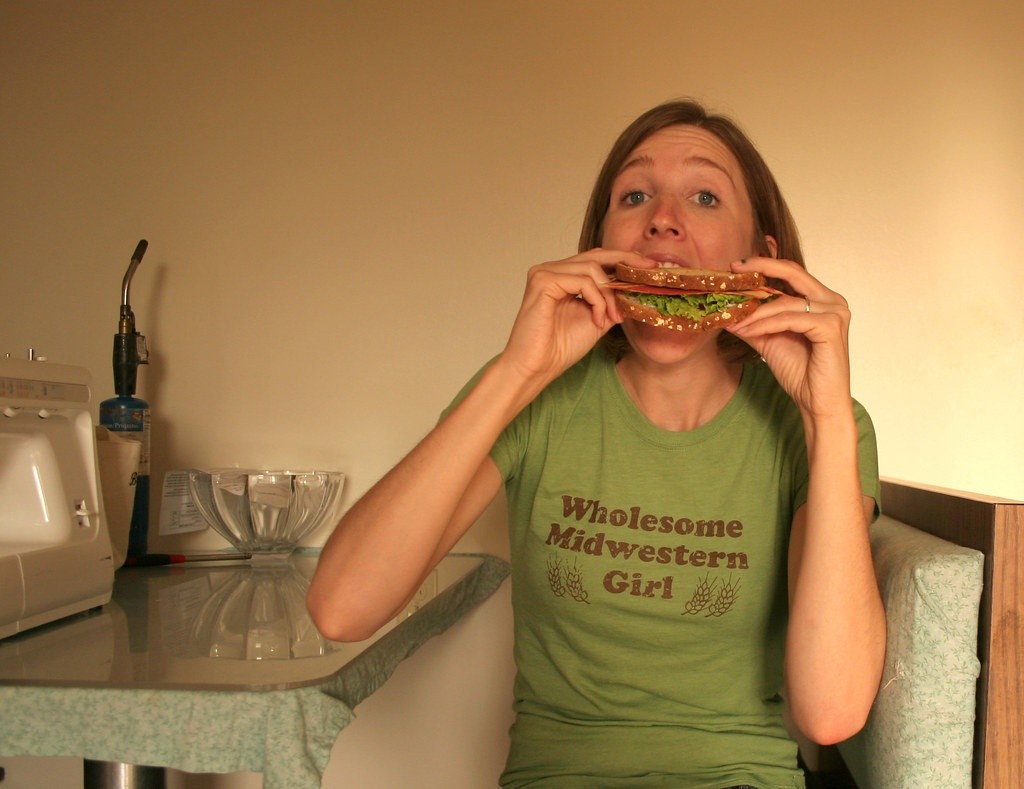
[299,98,890,789]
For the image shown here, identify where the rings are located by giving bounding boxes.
[803,296,810,312]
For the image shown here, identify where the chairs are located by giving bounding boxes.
[802,473,1023,789]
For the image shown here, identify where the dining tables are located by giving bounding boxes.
[0,549,513,789]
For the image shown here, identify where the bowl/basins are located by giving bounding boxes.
[188,468,348,554]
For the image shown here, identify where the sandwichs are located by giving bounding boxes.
[600,263,786,331]
